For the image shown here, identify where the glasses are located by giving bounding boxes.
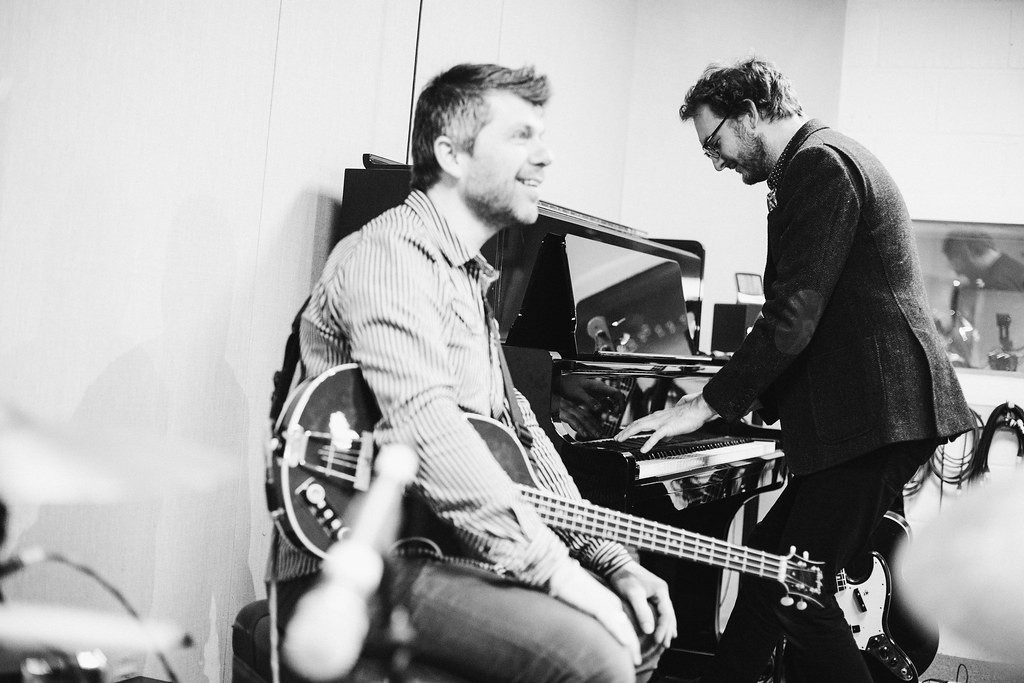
[702,98,738,158]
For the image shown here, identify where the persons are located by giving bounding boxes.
[943,229,1024,372]
[265,61,679,683]
[615,60,977,683]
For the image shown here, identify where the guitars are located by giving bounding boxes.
[268,364,940,683]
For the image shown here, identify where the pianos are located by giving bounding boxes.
[340,162,792,682]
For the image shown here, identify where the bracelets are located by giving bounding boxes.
[550,560,580,598]
